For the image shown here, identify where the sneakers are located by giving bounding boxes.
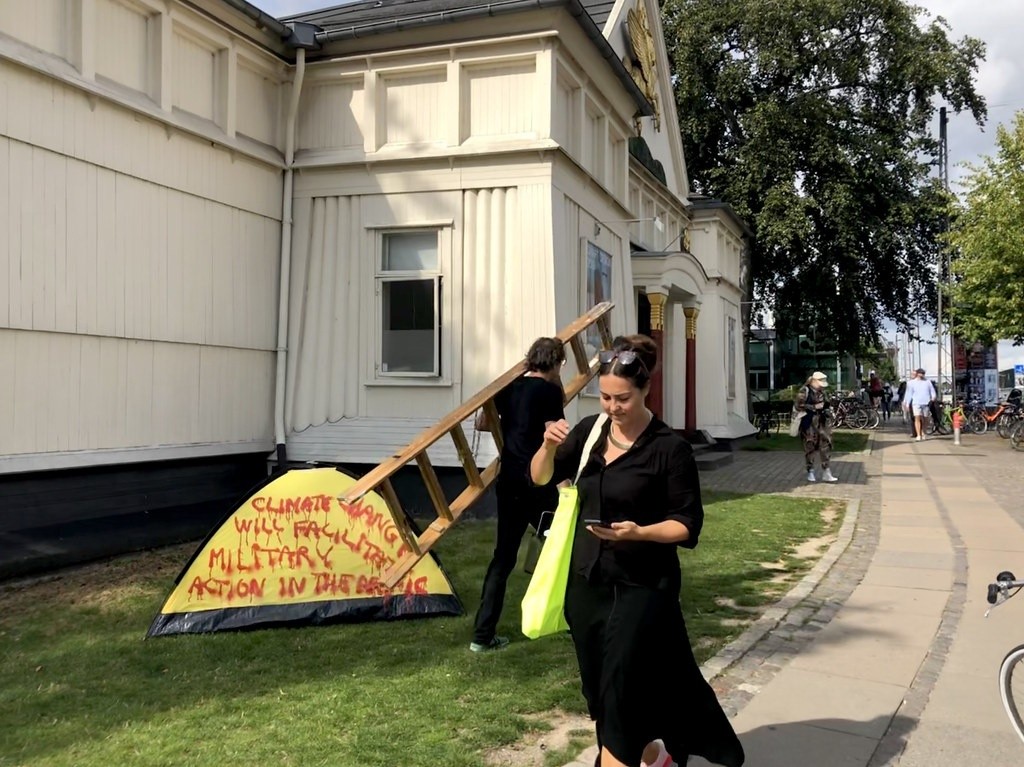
[807,469,816,481]
[469,635,509,652]
[822,468,838,481]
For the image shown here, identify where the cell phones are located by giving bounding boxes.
[583,519,613,529]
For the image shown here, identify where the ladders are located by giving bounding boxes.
[347,298,618,589]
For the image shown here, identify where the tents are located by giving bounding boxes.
[144,461,464,636]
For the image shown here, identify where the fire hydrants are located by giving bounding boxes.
[953,412,965,446]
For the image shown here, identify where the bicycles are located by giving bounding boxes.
[920,389,1024,452]
[750,387,782,442]
[985,572,1024,744]
[824,390,880,430]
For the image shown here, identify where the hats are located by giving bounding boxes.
[885,382,889,386]
[917,368,925,374]
[813,371,829,387]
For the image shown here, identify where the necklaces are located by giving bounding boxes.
[610,407,653,451]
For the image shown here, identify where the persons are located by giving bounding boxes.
[795,371,838,482]
[530,333,747,767]
[471,337,566,652]
[897,369,937,442]
[868,373,893,421]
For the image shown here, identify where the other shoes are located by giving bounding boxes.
[922,434,926,440]
[916,435,921,441]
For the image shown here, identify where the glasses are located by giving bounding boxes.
[597,349,652,379]
[561,355,567,366]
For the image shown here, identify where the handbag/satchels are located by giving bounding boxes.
[928,401,941,423]
[789,386,809,437]
[520,411,611,639]
